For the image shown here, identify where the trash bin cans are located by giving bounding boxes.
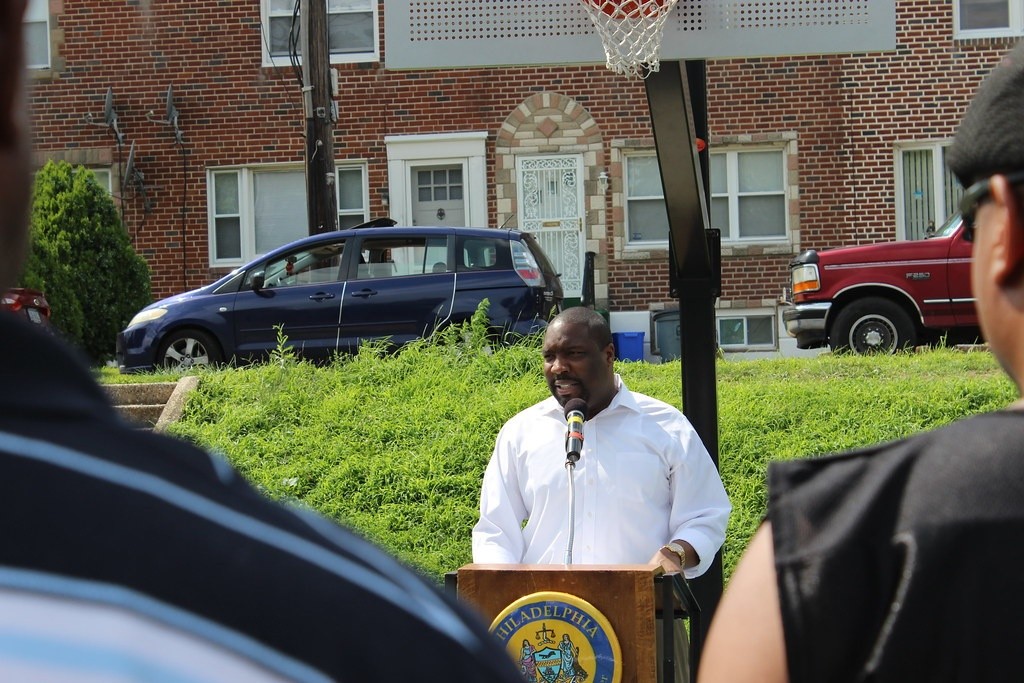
[612,332,645,364]
[649,301,681,365]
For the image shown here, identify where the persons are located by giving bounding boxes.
[470,305,731,579]
[696,37,1024,683]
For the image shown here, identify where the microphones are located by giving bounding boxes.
[564,398,591,461]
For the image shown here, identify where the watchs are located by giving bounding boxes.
[658,542,686,570]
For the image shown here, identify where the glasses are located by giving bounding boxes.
[958,179,998,241]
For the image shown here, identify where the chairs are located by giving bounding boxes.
[432,261,447,274]
[371,261,393,278]
[357,252,372,279]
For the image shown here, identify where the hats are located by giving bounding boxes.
[946,38,1024,180]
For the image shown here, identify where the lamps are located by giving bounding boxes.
[597,172,610,195]
[381,192,389,206]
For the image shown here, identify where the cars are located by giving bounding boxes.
[116,216,563,375]
[782,211,978,357]
[2,288,51,329]
